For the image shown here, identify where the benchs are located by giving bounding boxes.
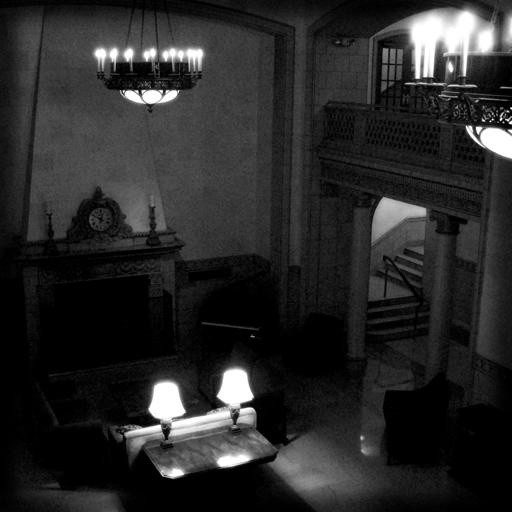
[199,349,286,434]
[32,373,104,430]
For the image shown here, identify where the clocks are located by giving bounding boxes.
[68,186,133,244]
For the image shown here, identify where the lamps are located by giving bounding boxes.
[148,379,186,448]
[93,0,204,114]
[404,0,512,159]
[215,366,253,434]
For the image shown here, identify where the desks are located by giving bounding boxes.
[143,424,278,482]
[110,380,148,417]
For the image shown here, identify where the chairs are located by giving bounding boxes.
[282,312,344,377]
[383,375,451,464]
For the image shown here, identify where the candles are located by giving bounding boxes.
[149,196,155,207]
[46,199,52,214]
